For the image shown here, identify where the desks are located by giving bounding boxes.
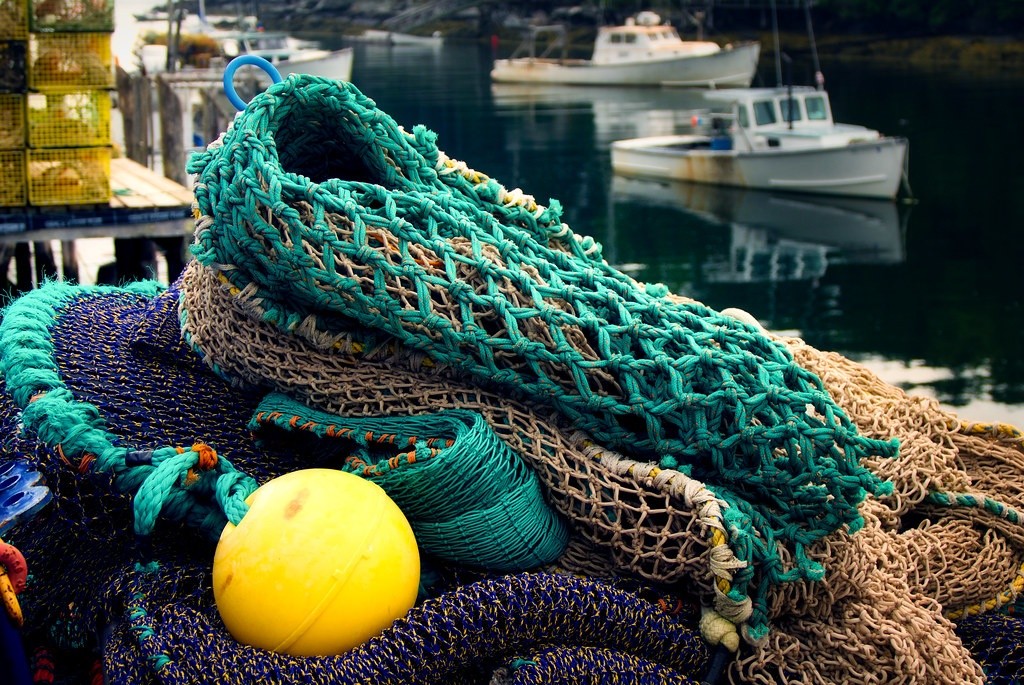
[1,157,197,299]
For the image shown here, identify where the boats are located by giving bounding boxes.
[606,0,912,200]
[340,27,446,46]
[486,9,764,90]
[98,0,358,148]
[607,174,908,265]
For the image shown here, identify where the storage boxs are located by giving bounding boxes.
[0,0,114,205]
